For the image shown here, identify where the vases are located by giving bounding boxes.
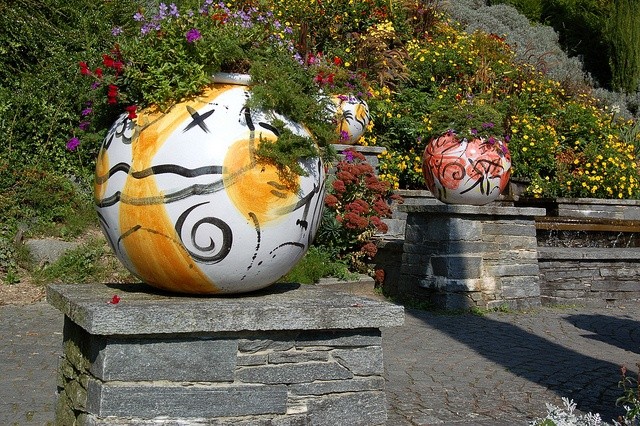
[423,133,511,204]
[96,75,326,295]
[314,94,370,144]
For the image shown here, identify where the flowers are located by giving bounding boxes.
[426,99,505,140]
[64,0,346,184]
[308,54,373,97]
